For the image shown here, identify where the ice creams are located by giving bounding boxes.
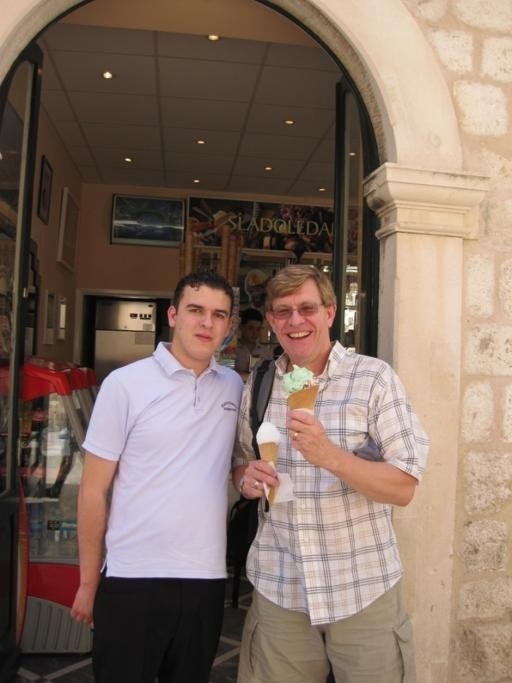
[281,364,319,411]
[256,422,283,505]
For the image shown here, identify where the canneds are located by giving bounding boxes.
[21,431,41,468]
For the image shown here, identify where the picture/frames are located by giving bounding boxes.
[55,182,81,275]
[35,151,54,226]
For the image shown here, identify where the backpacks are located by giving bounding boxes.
[225,353,277,575]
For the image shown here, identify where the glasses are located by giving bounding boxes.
[266,300,328,320]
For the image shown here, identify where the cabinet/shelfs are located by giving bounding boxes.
[189,243,298,378]
[298,249,361,357]
[73,364,103,419]
[18,352,94,656]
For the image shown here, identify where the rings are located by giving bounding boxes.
[254,481,259,489]
[293,432,298,440]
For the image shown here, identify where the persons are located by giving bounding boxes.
[233,265,430,683]
[69,270,245,683]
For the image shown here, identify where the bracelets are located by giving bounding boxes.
[238,478,244,492]
